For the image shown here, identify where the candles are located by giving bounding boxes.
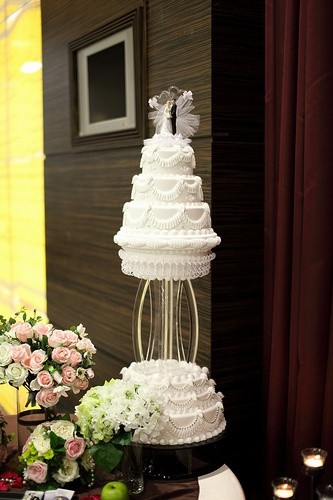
[273,478,297,500]
[301,448,328,492]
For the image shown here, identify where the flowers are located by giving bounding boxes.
[17,414,86,491]
[75,378,169,473]
[0,471,23,491]
[0,307,97,408]
[0,411,15,446]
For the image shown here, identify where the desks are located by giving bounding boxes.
[0,414,245,500]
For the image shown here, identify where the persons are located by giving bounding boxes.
[161,98,177,135]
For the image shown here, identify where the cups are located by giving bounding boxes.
[115,462,145,496]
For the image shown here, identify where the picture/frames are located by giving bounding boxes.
[68,6,147,154]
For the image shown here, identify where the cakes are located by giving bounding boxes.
[118,359,225,444]
[114,133,221,280]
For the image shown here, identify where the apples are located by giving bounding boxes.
[101,481,129,500]
